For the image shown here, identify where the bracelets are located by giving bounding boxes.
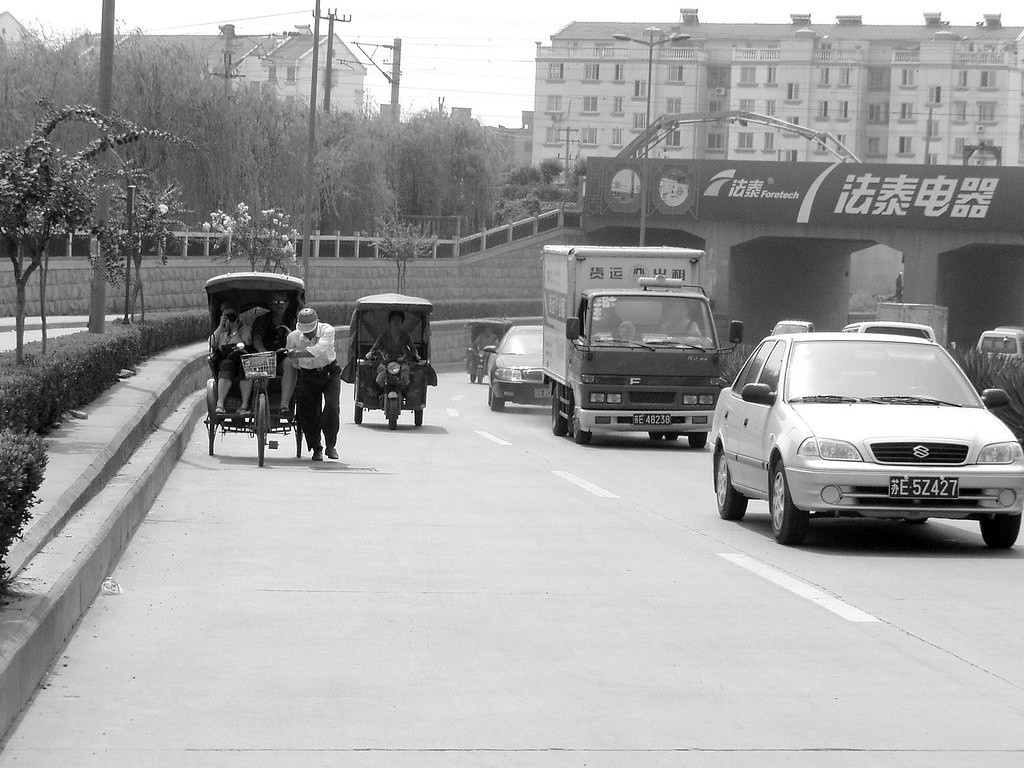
[230,332,237,337]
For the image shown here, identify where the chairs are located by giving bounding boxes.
[591,302,622,339]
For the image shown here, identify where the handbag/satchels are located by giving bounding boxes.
[221,342,252,359]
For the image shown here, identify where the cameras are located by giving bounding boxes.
[225,313,238,323]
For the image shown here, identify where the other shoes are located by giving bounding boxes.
[215,405,226,414]
[280,406,291,414]
[312,448,324,460]
[236,405,249,413]
[325,447,338,459]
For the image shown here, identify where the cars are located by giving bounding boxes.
[705,330,1024,552]
[483,325,554,412]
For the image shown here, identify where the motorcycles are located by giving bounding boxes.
[464,317,514,384]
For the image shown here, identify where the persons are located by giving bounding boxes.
[209,303,254,416]
[284,307,342,461]
[252,290,298,414]
[366,311,426,398]
[658,301,701,337]
[471,326,499,372]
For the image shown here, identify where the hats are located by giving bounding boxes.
[296,307,318,334]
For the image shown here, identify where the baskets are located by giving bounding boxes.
[240,352,277,379]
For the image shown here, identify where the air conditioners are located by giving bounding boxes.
[973,159,982,166]
[715,87,725,96]
[711,121,722,127]
[976,126,984,132]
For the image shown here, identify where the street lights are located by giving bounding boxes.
[612,32,692,246]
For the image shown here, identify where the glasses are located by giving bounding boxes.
[271,299,290,305]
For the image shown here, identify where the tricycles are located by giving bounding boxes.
[203,270,307,468]
[351,292,434,431]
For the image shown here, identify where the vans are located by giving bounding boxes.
[769,320,816,337]
[975,325,1024,364]
[840,320,937,344]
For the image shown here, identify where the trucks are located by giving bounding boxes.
[541,242,746,447]
